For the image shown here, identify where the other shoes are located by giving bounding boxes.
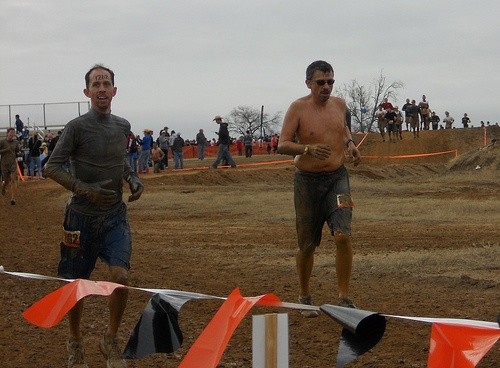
[10,199,16,206]
[39,177,45,180]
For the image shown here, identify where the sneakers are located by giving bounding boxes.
[336,297,356,309]
[298,295,318,317]
[98,330,126,368]
[67,337,88,368]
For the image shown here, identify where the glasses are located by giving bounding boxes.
[310,79,335,86]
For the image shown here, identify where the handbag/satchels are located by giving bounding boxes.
[28,150,32,161]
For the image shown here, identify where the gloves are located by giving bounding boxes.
[125,174,145,202]
[80,179,118,207]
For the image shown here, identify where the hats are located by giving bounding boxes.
[212,115,222,121]
[143,128,150,133]
[32,131,38,135]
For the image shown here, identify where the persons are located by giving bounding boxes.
[15,115,23,141]
[238,139,242,156]
[277,60,362,316]
[374,94,444,143]
[0,128,19,205]
[481,121,498,127]
[196,129,207,160]
[259,134,279,154]
[43,65,145,368]
[210,138,215,147]
[244,130,252,159]
[443,111,455,129]
[15,126,71,181]
[209,115,237,168]
[462,113,470,127]
[118,127,185,191]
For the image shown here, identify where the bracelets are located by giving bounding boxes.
[347,139,354,147]
[303,144,310,156]
